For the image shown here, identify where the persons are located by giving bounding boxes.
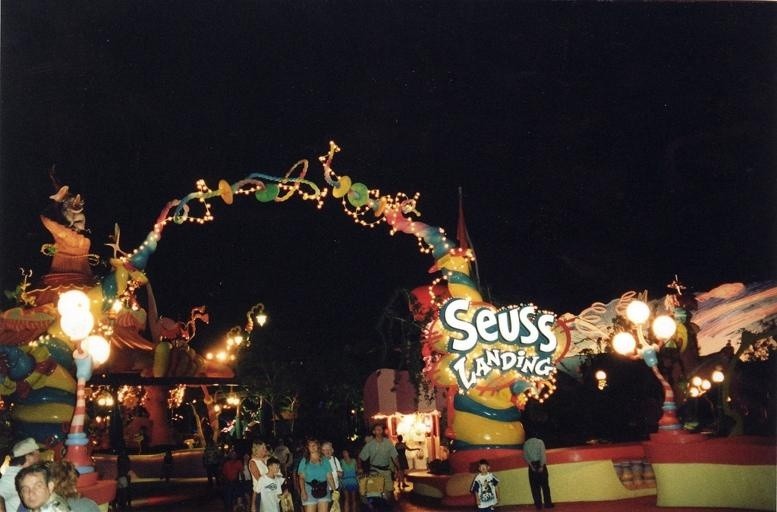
[35,459,80,511]
[469,458,501,511]
[521,428,555,510]
[0,436,50,511]
[13,463,72,511]
[199,422,420,511]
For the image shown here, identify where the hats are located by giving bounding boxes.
[12,437,48,459]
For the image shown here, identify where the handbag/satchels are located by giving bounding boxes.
[309,479,329,499]
